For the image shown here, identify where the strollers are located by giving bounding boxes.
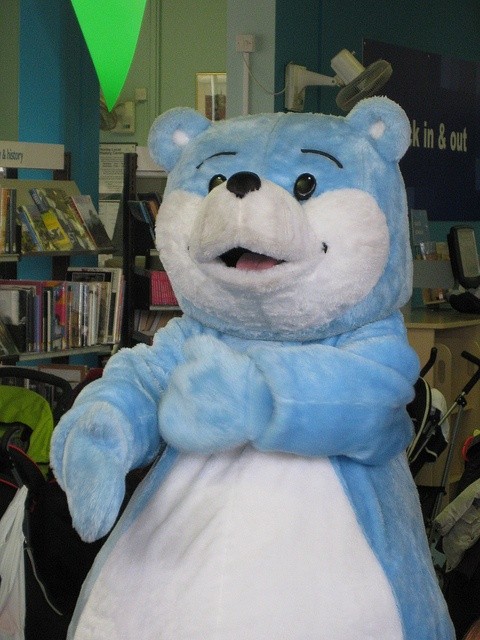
[406,346,480,591]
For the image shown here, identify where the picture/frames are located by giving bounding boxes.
[195,72,227,123]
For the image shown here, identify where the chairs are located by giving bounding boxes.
[0,366,72,450]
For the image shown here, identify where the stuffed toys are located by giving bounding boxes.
[49,97,457,640]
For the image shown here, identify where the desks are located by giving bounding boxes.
[405,308,480,510]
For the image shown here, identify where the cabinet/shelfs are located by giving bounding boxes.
[0,153,123,421]
[122,152,185,349]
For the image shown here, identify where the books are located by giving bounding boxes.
[40,363,89,391]
[0,187,116,254]
[132,308,177,337]
[134,253,178,308]
[127,192,162,252]
[0,268,125,354]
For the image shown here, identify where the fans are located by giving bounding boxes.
[284,48,393,116]
